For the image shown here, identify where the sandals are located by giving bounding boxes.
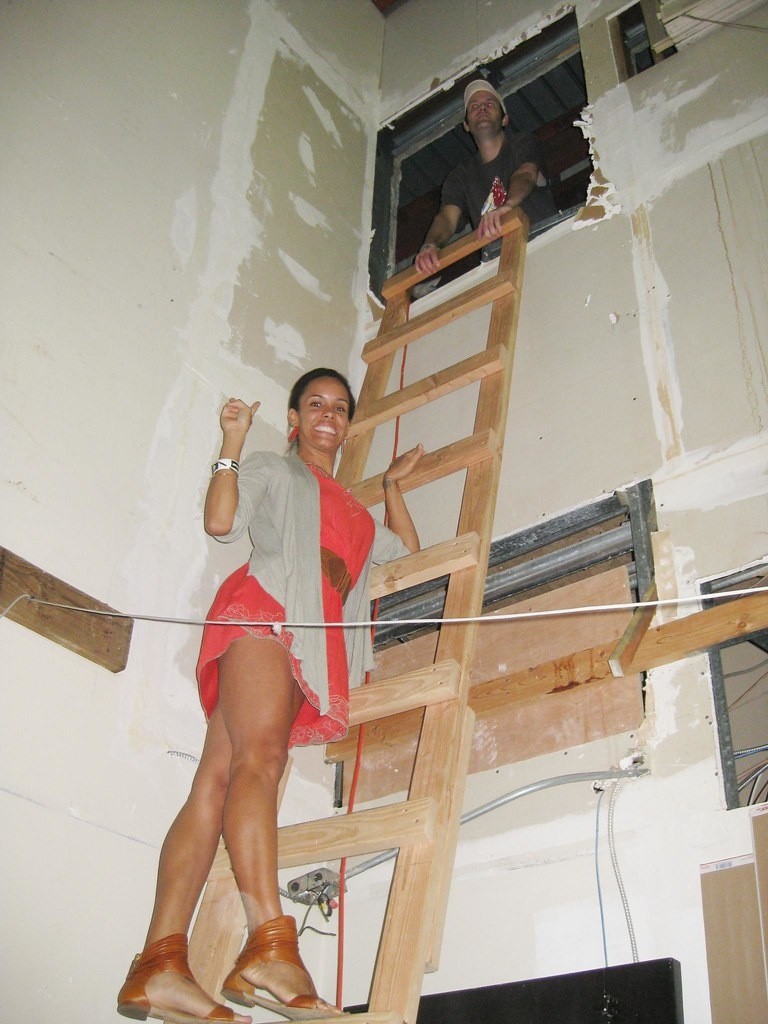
[118,933,253,1024]
[221,915,350,1021]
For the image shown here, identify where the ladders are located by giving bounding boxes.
[160,204,530,1024]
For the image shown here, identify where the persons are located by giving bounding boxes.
[414,79,558,263]
[116,367,427,1024]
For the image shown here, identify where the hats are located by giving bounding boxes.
[463,79,507,119]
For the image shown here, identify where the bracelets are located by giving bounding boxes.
[212,459,239,479]
[419,244,436,250]
